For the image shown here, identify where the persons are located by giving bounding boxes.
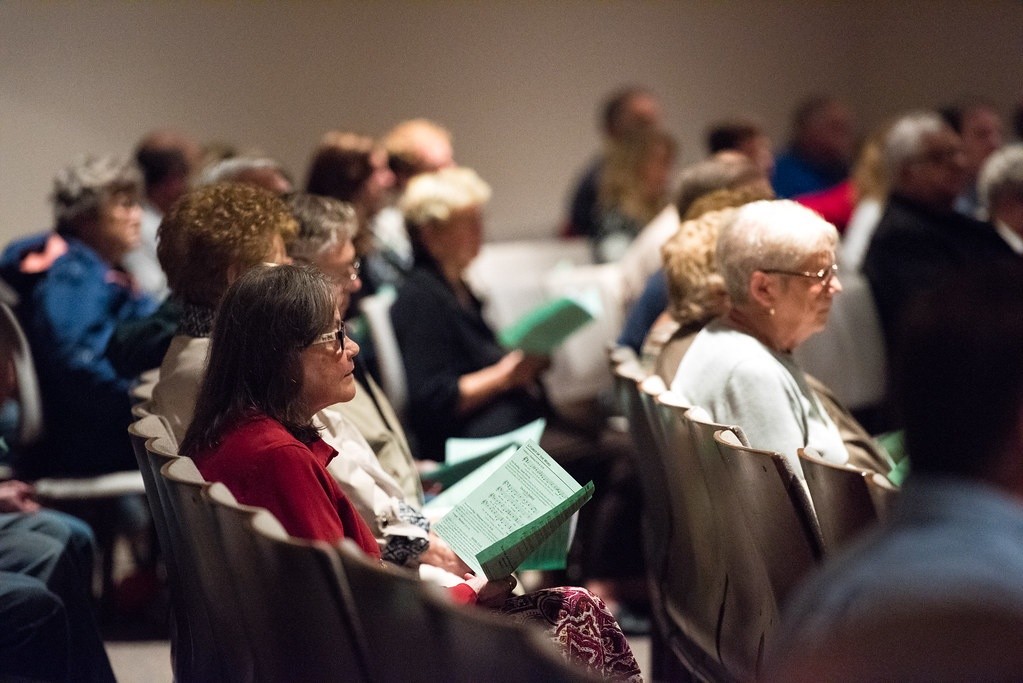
[177,260,647,682]
[668,194,901,509]
[0,84,1023,683]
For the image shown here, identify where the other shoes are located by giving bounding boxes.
[610,609,656,636]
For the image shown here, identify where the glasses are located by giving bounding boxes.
[331,254,361,289]
[296,320,346,351]
[759,264,839,286]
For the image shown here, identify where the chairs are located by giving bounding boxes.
[0,260,890,683]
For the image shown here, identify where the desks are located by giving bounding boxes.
[359,259,901,419]
